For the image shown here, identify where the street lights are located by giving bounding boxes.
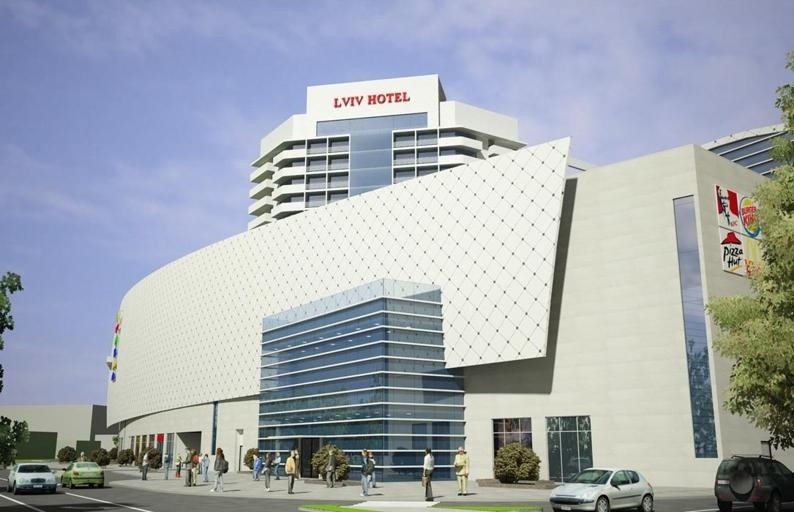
[234,427,244,471]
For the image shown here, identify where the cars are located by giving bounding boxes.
[549,466,654,511]
[60,461,104,487]
[6,462,57,494]
[345,455,364,469]
[568,456,590,472]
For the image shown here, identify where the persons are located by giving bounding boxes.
[423,447,435,499]
[325,449,337,487]
[250,448,300,494]
[142,451,149,481]
[360,449,376,496]
[164,447,226,492]
[454,447,469,496]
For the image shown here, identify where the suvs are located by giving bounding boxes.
[714,455,794,512]
